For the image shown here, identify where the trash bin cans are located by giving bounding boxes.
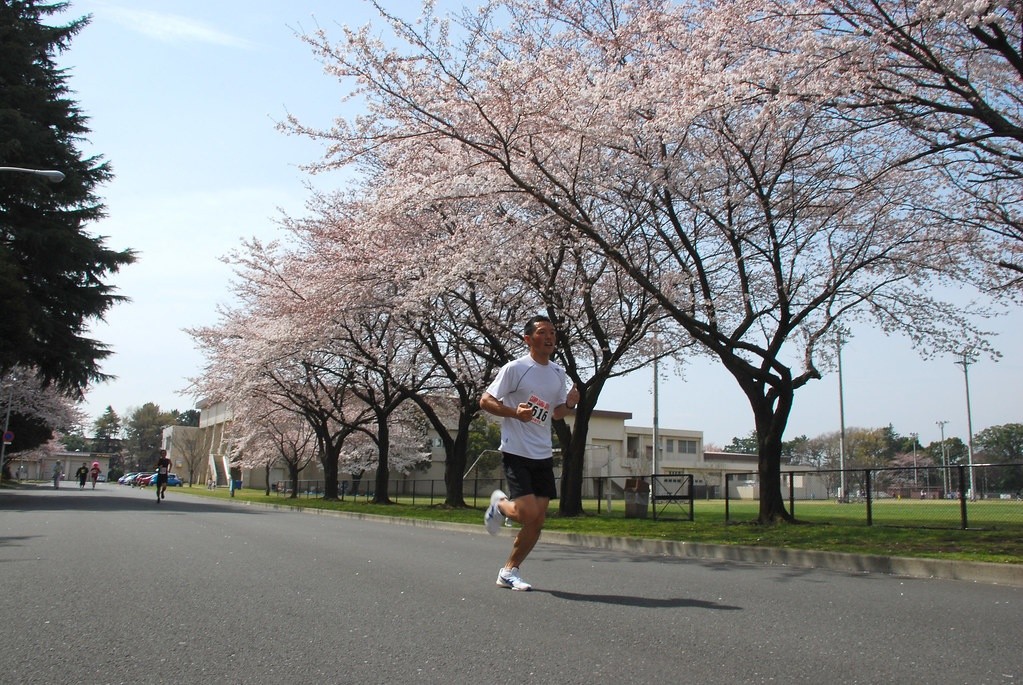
[623,478,650,520]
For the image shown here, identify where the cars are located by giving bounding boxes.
[117,471,184,488]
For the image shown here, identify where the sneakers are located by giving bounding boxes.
[484,490,508,538]
[496,567,532,591]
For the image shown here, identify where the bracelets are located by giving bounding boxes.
[566,401,575,409]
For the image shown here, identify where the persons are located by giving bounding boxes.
[51,459,65,489]
[153,450,172,503]
[892,492,896,497]
[861,488,865,496]
[75,463,89,490]
[89,462,101,490]
[479,315,580,591]
[947,488,961,499]
[921,488,925,500]
[1016,490,1021,501]
[208,478,212,490]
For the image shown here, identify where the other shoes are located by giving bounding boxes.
[160,491,164,498]
[157,498,160,502]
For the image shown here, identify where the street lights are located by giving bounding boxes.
[935,420,949,499]
[909,432,919,490]
[962,345,979,502]
[828,323,849,504]
